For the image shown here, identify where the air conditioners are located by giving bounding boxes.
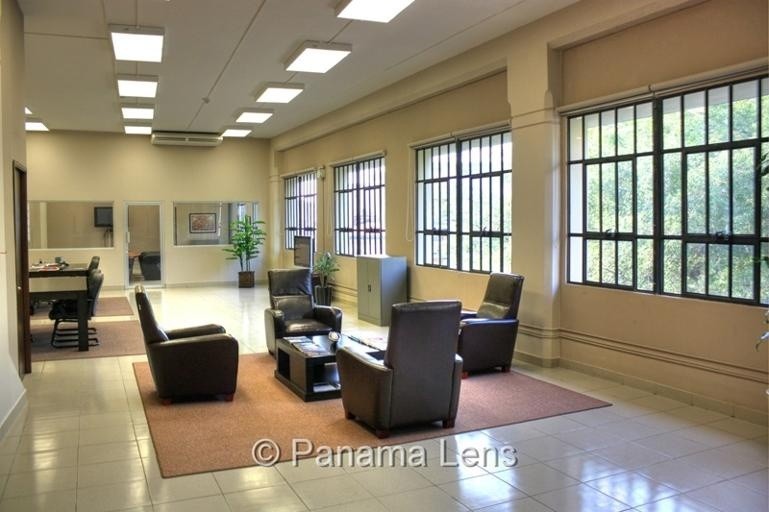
[150,132,223,148]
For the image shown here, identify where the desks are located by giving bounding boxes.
[29,262,88,345]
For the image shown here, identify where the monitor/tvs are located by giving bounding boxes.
[293,235,313,268]
[94,206,130,228]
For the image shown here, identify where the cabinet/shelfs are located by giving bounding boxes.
[356,254,407,327]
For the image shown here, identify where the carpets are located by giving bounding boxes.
[132,352,614,479]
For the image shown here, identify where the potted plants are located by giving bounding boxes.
[312,252,340,306]
[222,214,266,288]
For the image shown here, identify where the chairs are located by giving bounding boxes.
[49,273,104,348]
[54,268,102,335]
[87,256,100,271]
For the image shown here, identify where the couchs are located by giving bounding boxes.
[264,268,342,358]
[336,300,464,437]
[458,272,524,378]
[135,286,239,406]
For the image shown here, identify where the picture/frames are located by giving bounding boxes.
[189,213,216,233]
